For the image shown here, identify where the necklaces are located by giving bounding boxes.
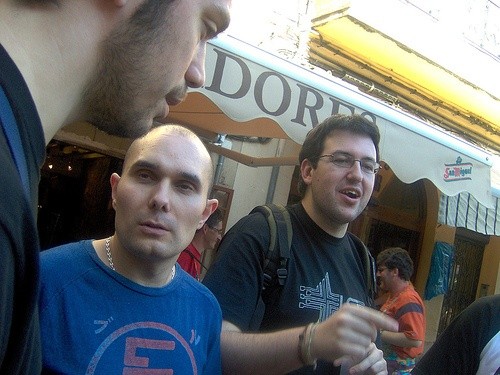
[104,237,176,287]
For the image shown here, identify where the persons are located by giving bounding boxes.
[372,247,425,375]
[32,124,224,375]
[0,1,233,375]
[409,294,500,375]
[199,113,400,375]
[178,205,224,281]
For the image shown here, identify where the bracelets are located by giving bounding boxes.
[296,319,318,370]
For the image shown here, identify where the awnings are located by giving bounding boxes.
[205,35,499,210]
[438,184,499,237]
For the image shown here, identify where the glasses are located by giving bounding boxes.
[207,228,224,234]
[313,152,382,174]
[376,267,393,273]
